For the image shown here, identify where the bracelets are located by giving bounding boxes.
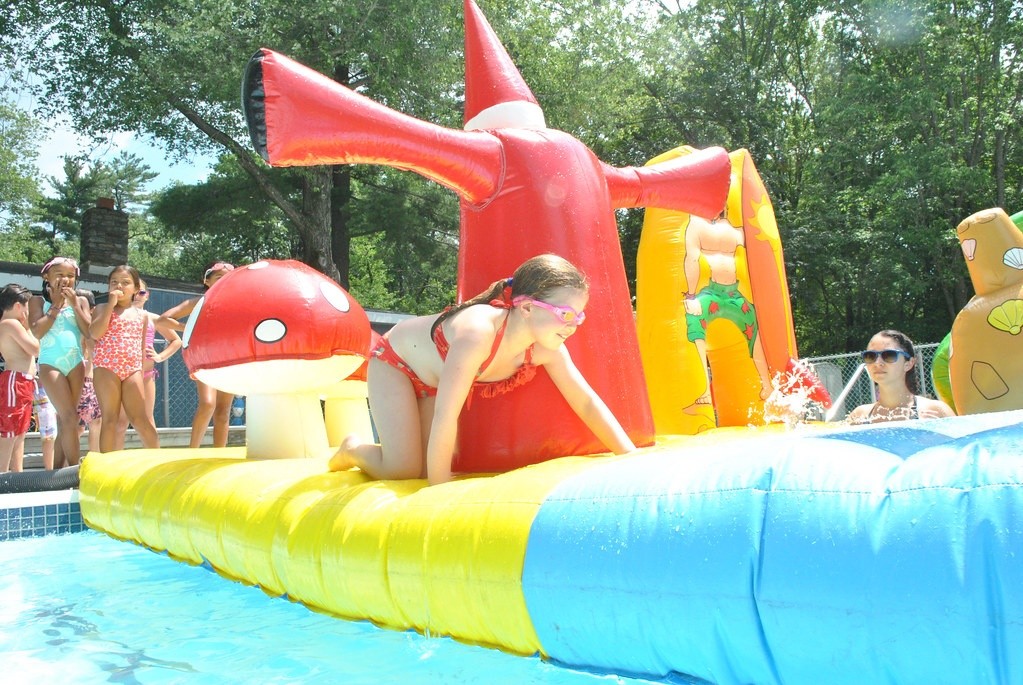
[45,313,56,321]
[50,304,60,312]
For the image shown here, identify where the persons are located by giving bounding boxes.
[847,330,956,425]
[325,252,636,482]
[0,255,182,472]
[156,262,235,449]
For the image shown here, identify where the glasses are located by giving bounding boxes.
[204,264,235,282]
[861,349,913,363]
[513,296,586,327]
[41,257,79,273]
[135,290,148,297]
